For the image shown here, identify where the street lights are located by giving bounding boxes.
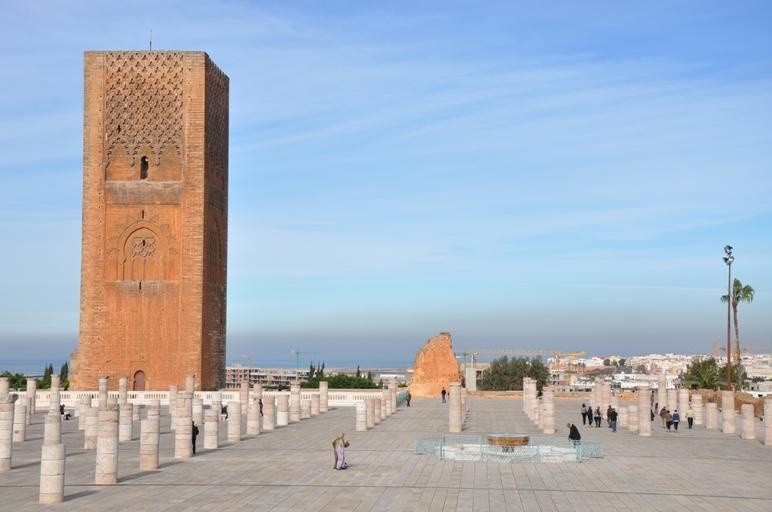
[725,245,734,391]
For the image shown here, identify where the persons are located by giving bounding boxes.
[594,406,602,428]
[654,401,659,414]
[581,403,588,425]
[259,399,263,416]
[192,420,201,455]
[59,404,72,421]
[331,433,350,469]
[685,406,696,430]
[405,390,412,407]
[597,407,602,422]
[567,422,581,448]
[440,385,447,404]
[611,408,618,433]
[587,407,594,426]
[607,404,613,428]
[651,408,656,432]
[664,410,673,433]
[672,410,681,432]
[222,405,228,420]
[660,405,669,427]
[335,433,346,471]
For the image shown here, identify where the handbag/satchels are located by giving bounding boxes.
[669,420,673,425]
